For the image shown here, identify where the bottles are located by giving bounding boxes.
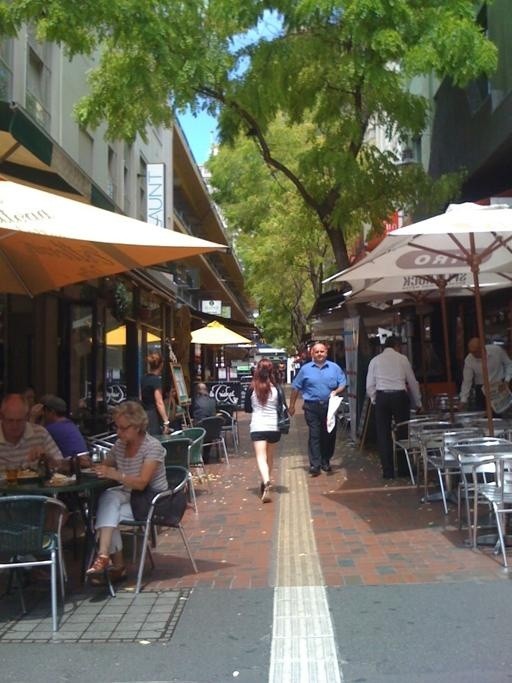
[36,453,50,486]
[70,450,82,481]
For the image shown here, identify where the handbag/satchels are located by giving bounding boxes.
[131,484,187,523]
[276,385,290,434]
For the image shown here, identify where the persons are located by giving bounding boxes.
[365,336,427,478]
[163,383,187,432]
[1,392,65,588]
[18,383,37,417]
[458,337,511,416]
[189,382,218,462]
[140,354,171,435]
[77,400,169,585]
[244,357,286,502]
[288,343,351,473]
[29,393,91,471]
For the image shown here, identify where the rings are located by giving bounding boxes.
[98,471,102,474]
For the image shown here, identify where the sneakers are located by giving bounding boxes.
[91,567,127,585]
[310,465,320,473]
[323,464,332,471]
[85,554,113,575]
[261,480,272,503]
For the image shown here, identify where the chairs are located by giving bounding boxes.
[152,427,215,495]
[0,496,71,631]
[86,466,198,594]
[189,415,232,469]
[389,394,512,570]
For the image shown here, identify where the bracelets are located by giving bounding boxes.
[163,420,170,425]
[117,471,127,484]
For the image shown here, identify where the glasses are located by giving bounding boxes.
[111,421,131,431]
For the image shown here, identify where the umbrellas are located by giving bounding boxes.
[89,323,162,351]
[321,203,511,437]
[190,320,252,381]
[311,300,403,355]
[0,173,228,298]
[342,265,511,421]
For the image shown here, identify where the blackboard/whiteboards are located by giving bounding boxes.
[357,397,371,453]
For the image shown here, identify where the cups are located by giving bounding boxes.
[92,447,109,465]
[5,468,19,486]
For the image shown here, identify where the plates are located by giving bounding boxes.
[80,466,116,476]
[6,473,40,479]
